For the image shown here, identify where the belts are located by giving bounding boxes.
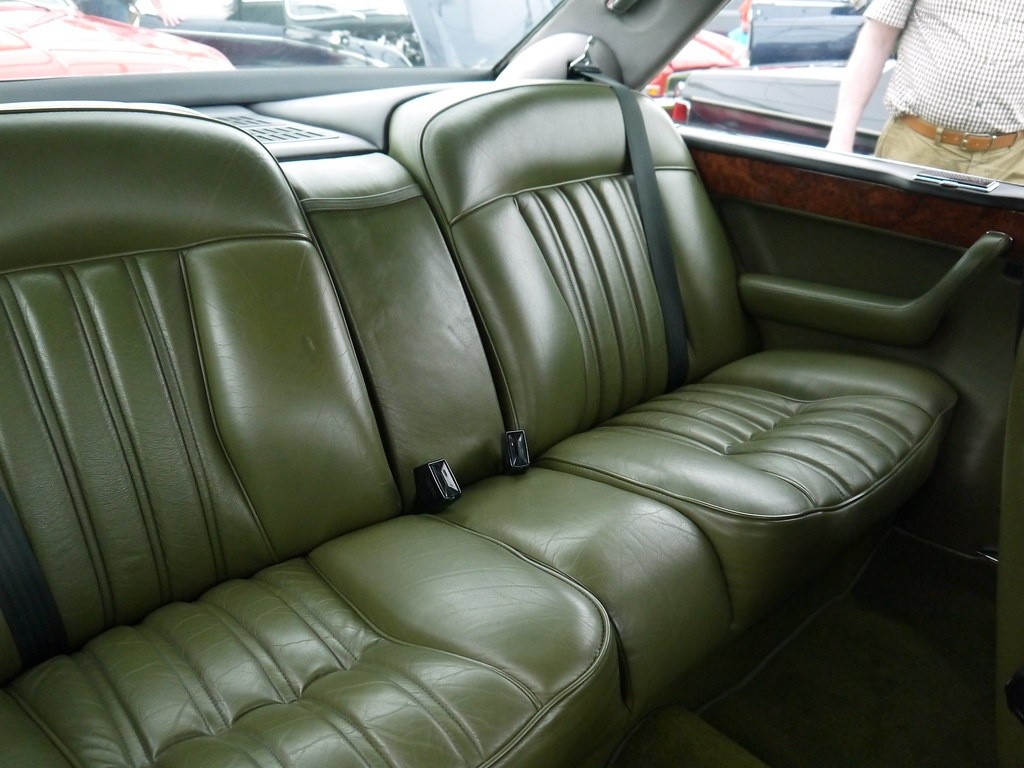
[899,114,1024,154]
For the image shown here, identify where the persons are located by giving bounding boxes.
[73,0,183,27]
[825,0,1024,185]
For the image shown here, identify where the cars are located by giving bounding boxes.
[0,1,426,83]
[629,1,899,158]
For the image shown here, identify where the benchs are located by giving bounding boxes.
[0,81,963,767]
